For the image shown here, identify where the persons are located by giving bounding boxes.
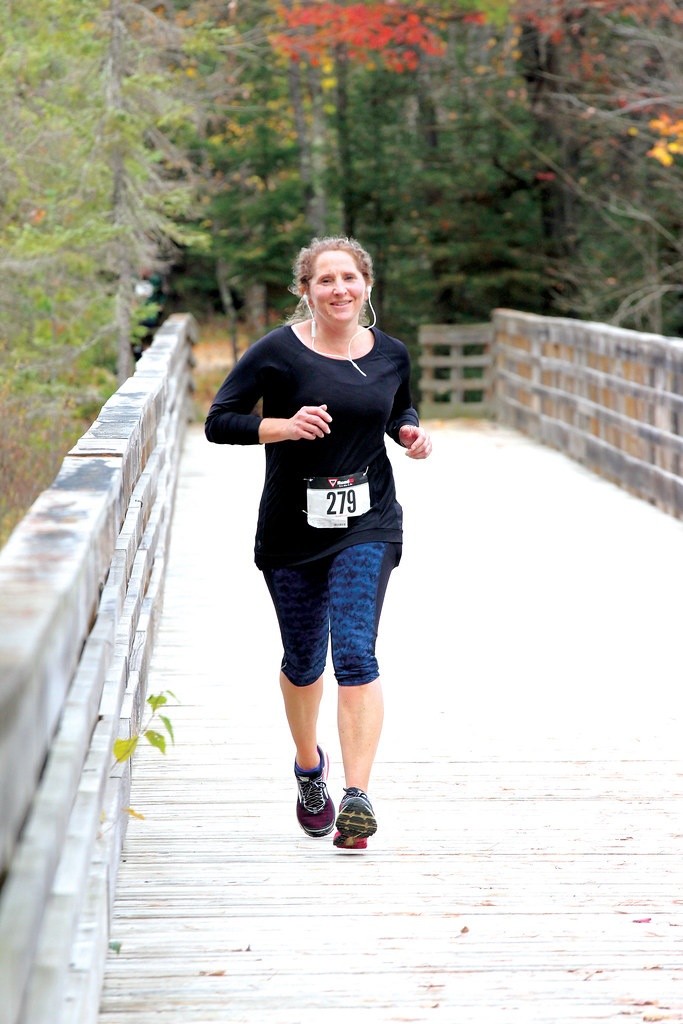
[205,236,431,849]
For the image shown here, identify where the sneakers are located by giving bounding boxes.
[334,787,377,849]
[293,742,335,838]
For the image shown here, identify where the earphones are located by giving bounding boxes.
[302,291,309,303]
[364,285,372,296]
